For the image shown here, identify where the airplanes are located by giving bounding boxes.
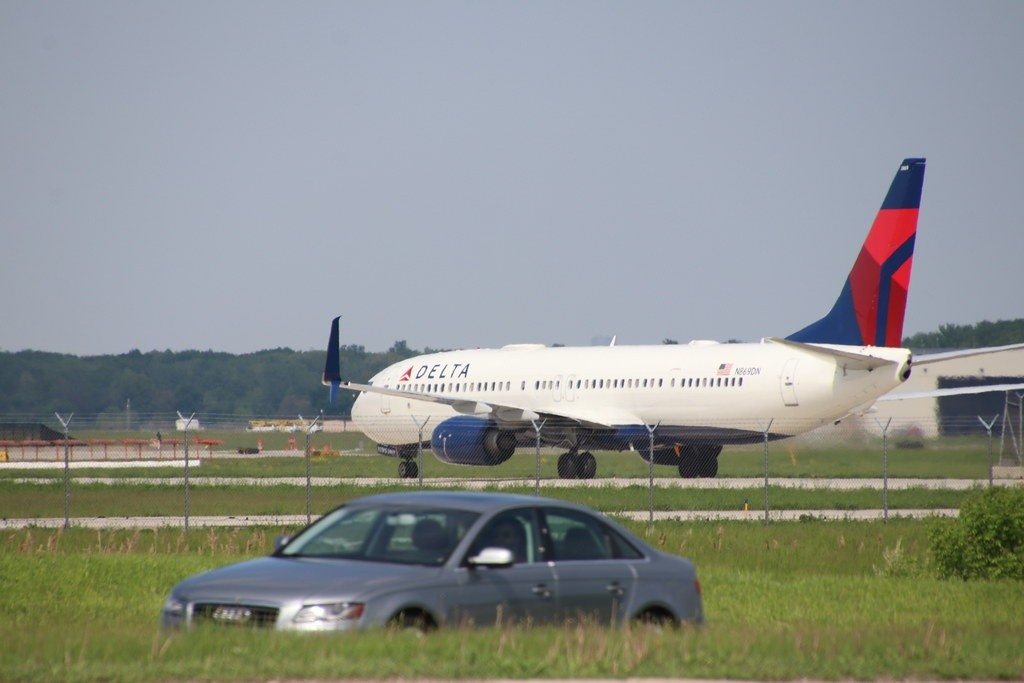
[321,158,1024,477]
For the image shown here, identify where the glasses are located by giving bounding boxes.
[492,529,514,539]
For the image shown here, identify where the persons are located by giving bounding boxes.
[483,519,528,564]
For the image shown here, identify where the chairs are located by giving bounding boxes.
[396,518,453,567]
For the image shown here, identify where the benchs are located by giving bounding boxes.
[553,527,608,560]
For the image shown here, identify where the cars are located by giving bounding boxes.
[164,487,706,643]
